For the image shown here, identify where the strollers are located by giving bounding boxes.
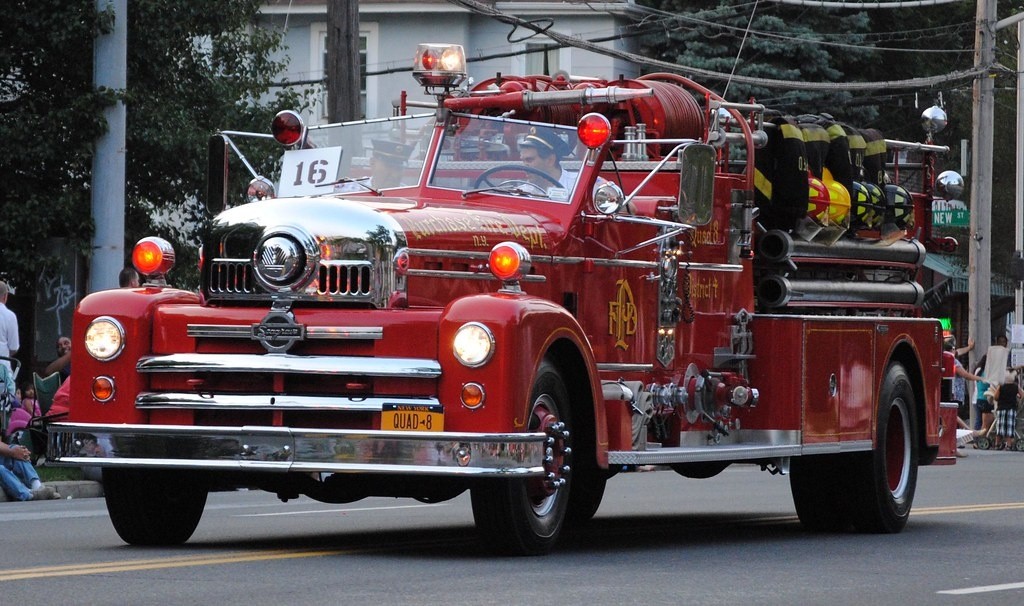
[976,379,1024,452]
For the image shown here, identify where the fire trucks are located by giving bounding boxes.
[45,44,959,555]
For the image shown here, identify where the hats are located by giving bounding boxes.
[371,138,413,165]
[519,125,570,162]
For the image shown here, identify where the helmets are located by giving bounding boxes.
[849,179,872,225]
[820,166,852,222]
[881,182,915,223]
[804,168,829,220]
[861,178,887,225]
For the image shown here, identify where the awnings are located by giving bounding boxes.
[922,253,1017,299]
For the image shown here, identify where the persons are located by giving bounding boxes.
[944,334,1024,451]
[508,126,638,215]
[332,137,415,195]
[0,280,104,500]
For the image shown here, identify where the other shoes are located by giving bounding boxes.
[995,444,1003,450]
[35,458,46,467]
[973,443,979,449]
[1006,446,1017,451]
[28,486,53,500]
[52,492,61,499]
[958,445,965,449]
